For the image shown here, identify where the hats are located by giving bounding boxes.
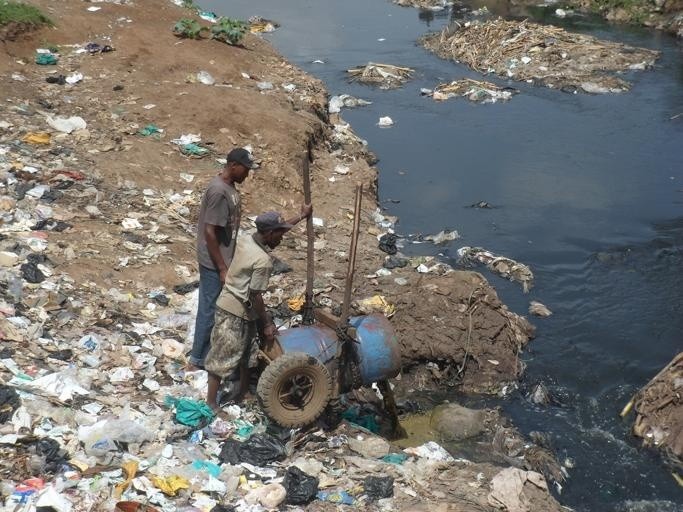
[226,148,255,169]
[254,212,293,231]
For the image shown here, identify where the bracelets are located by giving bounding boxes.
[299,212,302,223]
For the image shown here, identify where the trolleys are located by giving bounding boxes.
[251,148,405,436]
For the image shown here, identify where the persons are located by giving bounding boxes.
[203,201,314,421]
[189,148,253,371]
